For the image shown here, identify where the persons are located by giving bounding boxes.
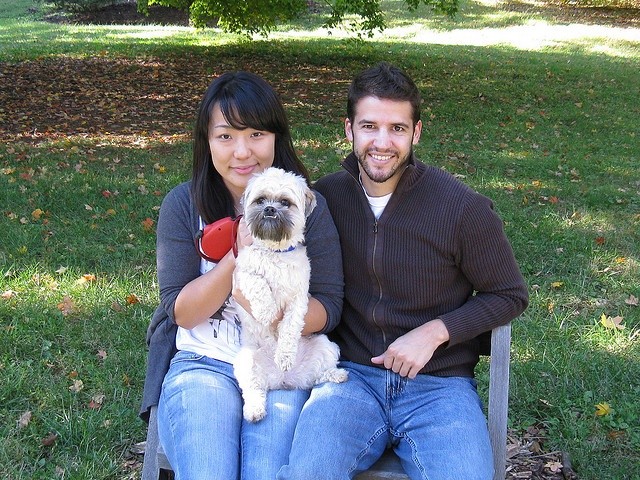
[138,70,347,480]
[276,63,529,480]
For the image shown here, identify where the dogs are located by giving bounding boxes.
[232,167,350,422]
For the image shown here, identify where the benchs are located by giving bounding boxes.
[141,318,513,480]
